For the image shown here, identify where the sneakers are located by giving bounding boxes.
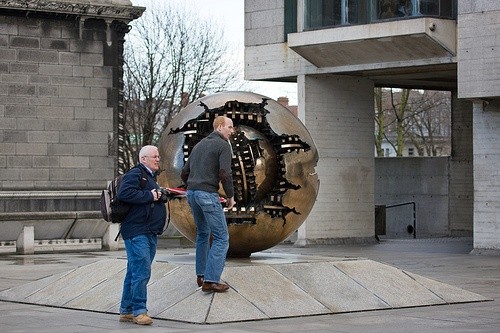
[133,314,153,324]
[120,313,133,322]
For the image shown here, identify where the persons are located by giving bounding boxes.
[116,145,170,326]
[181,116,236,293]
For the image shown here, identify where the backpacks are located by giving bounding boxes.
[100,166,147,223]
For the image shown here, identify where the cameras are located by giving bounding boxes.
[156,189,168,202]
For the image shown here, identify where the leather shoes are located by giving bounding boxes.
[196,276,204,287]
[203,282,230,292]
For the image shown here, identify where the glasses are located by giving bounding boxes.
[143,155,162,159]
